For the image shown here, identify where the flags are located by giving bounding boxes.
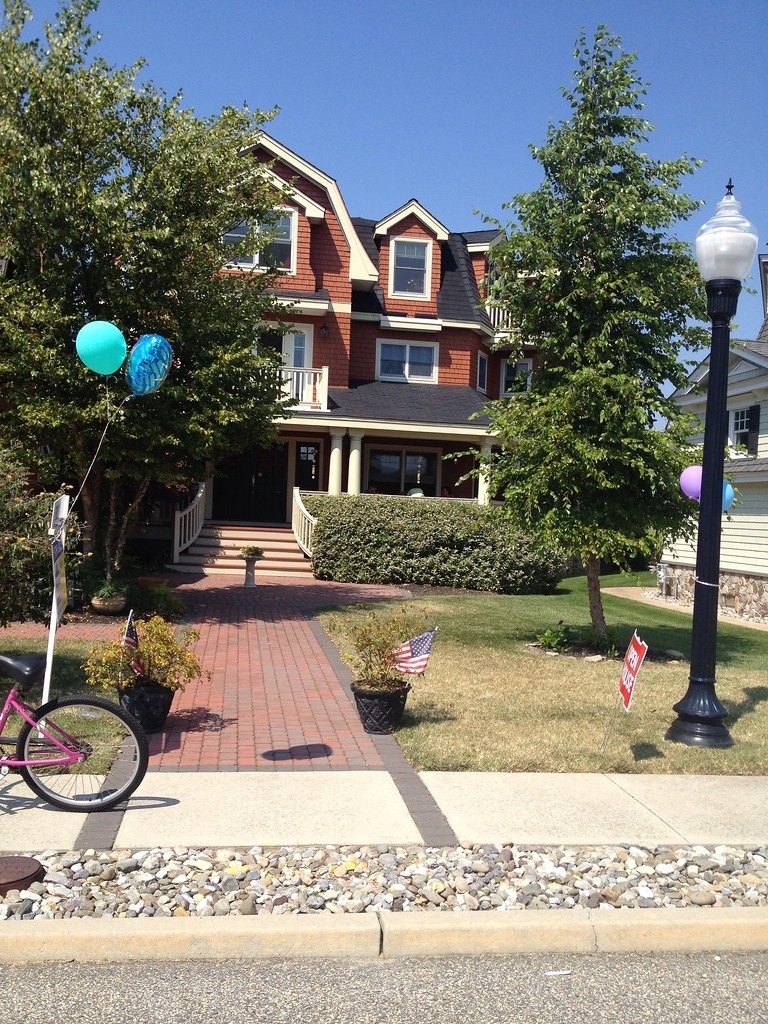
[382,630,435,675]
[120,610,145,676]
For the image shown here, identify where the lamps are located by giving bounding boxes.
[320,324,329,338]
[416,455,423,483]
[311,449,318,478]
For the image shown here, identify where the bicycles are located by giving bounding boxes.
[0,650,150,814]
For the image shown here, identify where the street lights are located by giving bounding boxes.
[666,174,759,748]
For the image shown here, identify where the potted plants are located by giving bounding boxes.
[238,545,266,587]
[78,614,214,735]
[321,604,439,734]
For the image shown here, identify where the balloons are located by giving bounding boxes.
[76,321,127,375]
[722,480,734,512]
[680,466,702,503]
[125,334,172,395]
